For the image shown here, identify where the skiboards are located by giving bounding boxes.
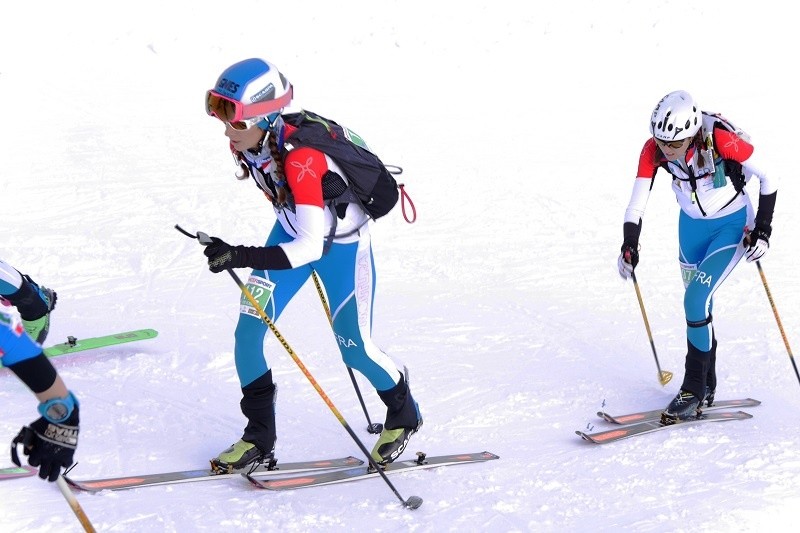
[0,328,159,480]
[63,451,501,492]
[574,398,762,445]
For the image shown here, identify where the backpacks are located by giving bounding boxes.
[655,111,755,182]
[282,111,398,219]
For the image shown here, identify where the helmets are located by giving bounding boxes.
[649,90,702,141]
[215,58,290,119]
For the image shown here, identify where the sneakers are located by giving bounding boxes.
[217,434,276,475]
[703,383,717,404]
[664,390,706,419]
[367,406,423,472]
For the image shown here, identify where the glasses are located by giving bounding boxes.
[204,89,245,123]
[225,119,261,130]
[652,138,685,148]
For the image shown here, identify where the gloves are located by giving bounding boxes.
[23,404,81,482]
[742,227,770,263]
[617,247,640,280]
[202,234,247,274]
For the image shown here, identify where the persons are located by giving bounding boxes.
[0,254,82,483]
[201,58,426,477]
[618,90,779,419]
